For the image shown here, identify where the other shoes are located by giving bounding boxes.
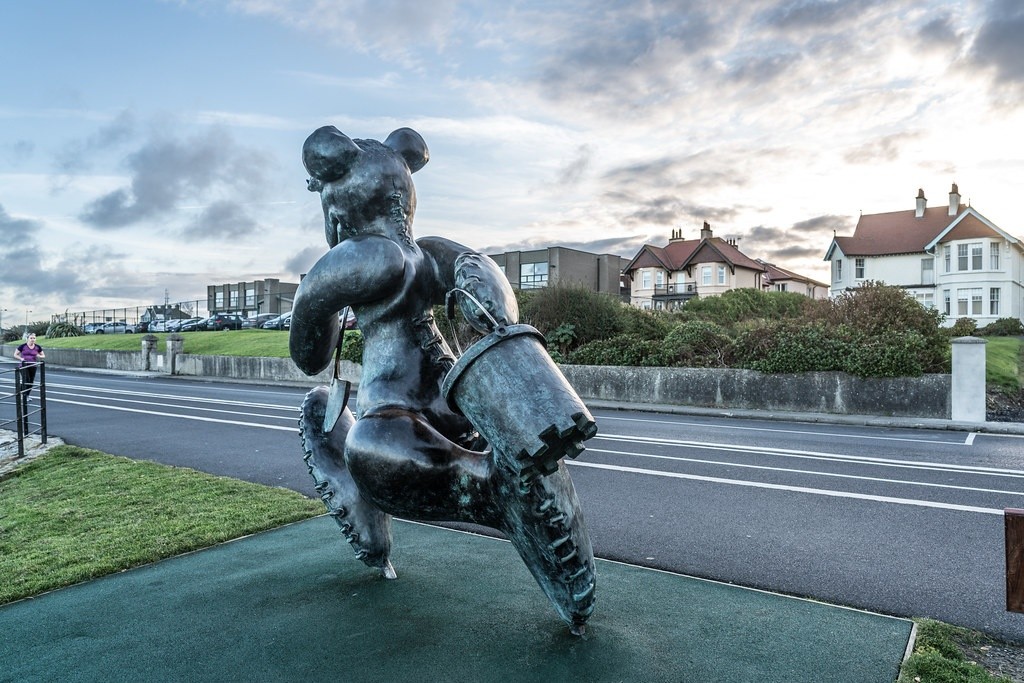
[27,396,32,402]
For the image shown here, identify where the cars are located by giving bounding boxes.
[80,310,359,336]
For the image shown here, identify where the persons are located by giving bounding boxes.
[290,125,520,522]
[14,333,45,402]
